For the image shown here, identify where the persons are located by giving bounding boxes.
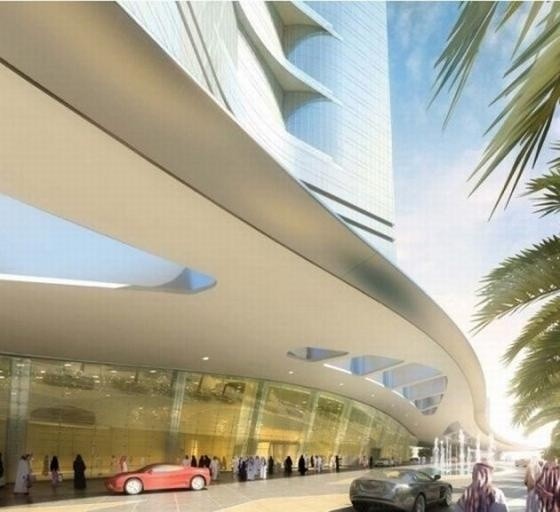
[181,453,219,481]
[524,458,547,512]
[120,456,129,474]
[12,454,35,495]
[535,460,560,512]
[335,455,343,470]
[453,461,510,511]
[232,453,321,481]
[73,454,86,489]
[369,455,374,468]
[50,455,61,485]
[0,453,3,479]
[391,457,396,466]
[360,455,368,469]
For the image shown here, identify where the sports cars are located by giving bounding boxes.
[349,467,453,511]
[27,402,96,426]
[104,461,211,495]
[40,365,102,391]
